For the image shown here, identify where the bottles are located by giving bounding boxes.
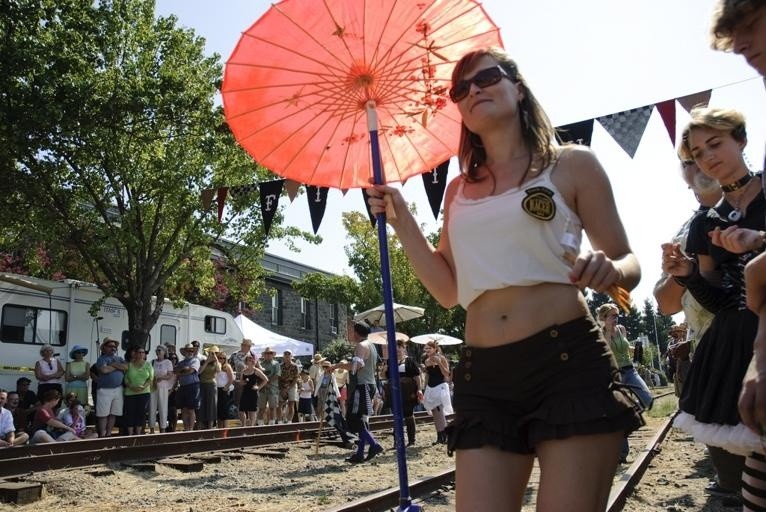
[634,337,642,363]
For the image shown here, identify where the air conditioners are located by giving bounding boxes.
[329,326,337,334]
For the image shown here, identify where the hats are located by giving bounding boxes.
[319,361,331,366]
[101,337,119,351]
[311,354,327,363]
[180,339,292,360]
[350,320,371,336]
[70,345,88,359]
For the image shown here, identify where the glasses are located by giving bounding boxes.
[449,66,513,103]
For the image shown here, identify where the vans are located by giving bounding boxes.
[0,271,248,410]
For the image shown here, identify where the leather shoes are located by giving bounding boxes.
[345,453,364,462]
[364,444,383,461]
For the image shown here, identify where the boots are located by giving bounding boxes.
[405,415,415,447]
[432,431,447,446]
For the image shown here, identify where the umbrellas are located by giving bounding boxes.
[354,301,425,325]
[219,0,507,500]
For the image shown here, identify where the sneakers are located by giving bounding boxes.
[705,481,736,496]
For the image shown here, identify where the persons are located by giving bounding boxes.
[366,48,641,512]
[0,320,466,464]
[651,0,766,512]
[597,303,653,464]
